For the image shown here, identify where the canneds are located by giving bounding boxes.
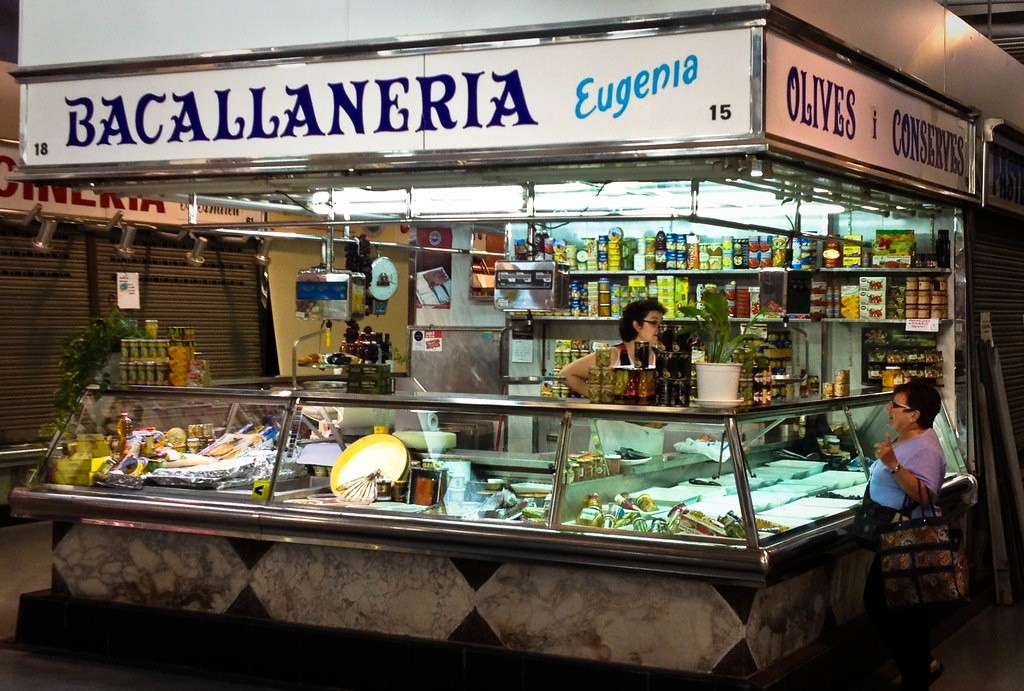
[745,330,792,398]
[724,284,736,317]
[117,320,197,386]
[375,479,409,502]
[883,366,904,388]
[92,458,116,481]
[807,375,819,391]
[826,286,842,318]
[827,438,840,454]
[567,456,607,484]
[588,341,691,407]
[516,231,687,317]
[905,276,948,320]
[550,349,589,398]
[576,490,748,541]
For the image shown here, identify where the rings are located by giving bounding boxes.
[878,449,881,454]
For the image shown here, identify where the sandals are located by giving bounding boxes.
[890,658,944,691]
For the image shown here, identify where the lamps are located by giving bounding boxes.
[112,227,136,257]
[185,233,207,266]
[254,237,272,261]
[31,219,58,253]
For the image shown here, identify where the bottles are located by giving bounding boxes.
[117,412,133,460]
[580,492,789,540]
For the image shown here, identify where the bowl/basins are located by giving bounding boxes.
[328,433,411,501]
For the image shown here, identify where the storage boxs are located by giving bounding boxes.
[859,276,887,320]
[348,361,395,393]
[869,229,917,268]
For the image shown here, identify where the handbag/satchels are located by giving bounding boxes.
[851,485,906,551]
[875,478,970,609]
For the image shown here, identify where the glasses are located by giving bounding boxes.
[639,319,660,327]
[890,400,913,411]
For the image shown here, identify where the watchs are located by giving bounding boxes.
[891,465,901,474]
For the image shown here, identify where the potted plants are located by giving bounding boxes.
[674,289,774,408]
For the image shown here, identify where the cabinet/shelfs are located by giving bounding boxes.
[531,265,952,400]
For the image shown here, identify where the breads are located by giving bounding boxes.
[297,352,322,364]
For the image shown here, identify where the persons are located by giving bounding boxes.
[558,300,666,452]
[867,379,947,691]
